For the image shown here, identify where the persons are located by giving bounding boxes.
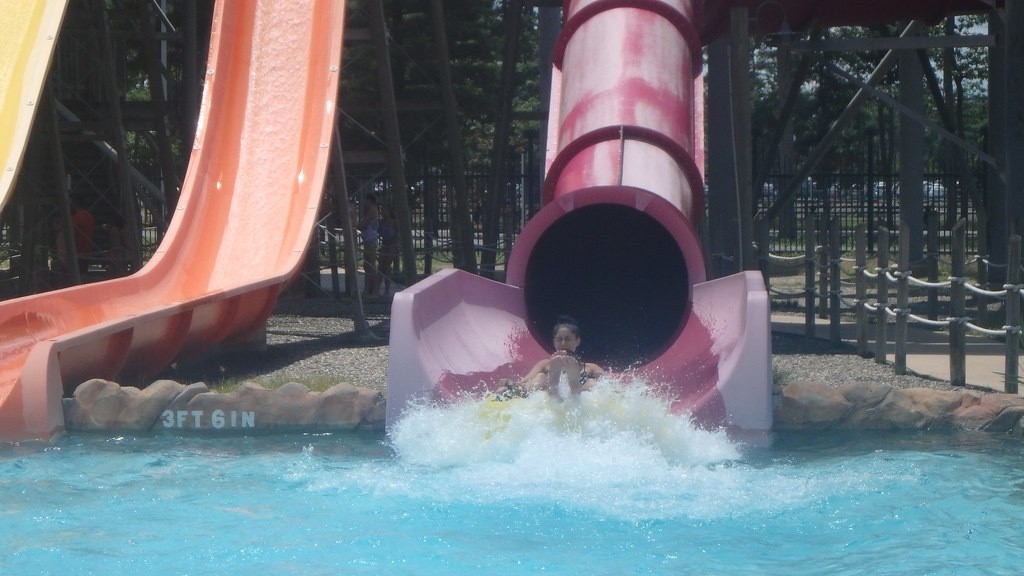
[377,204,400,296]
[495,316,606,403]
[57,199,94,286]
[355,192,379,294]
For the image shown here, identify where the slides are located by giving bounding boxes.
[0,0,345,447]
[381,0,776,434]
